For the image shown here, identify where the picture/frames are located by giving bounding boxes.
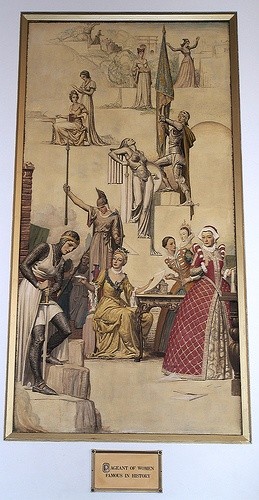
[2,10,252,446]
